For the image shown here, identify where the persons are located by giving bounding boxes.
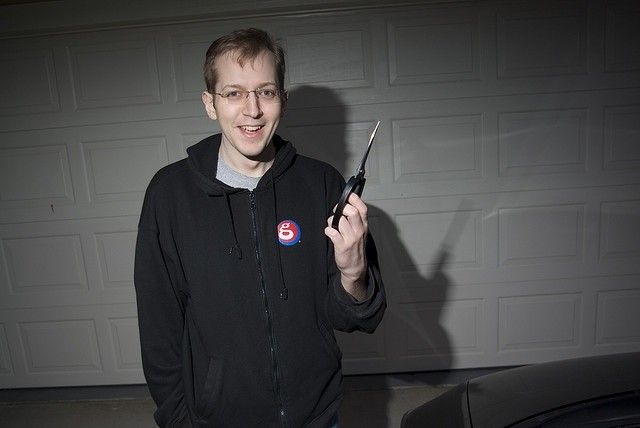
[132,27,388,426]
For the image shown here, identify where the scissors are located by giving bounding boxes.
[330,122,382,231]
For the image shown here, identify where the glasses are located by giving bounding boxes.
[208,88,285,103]
